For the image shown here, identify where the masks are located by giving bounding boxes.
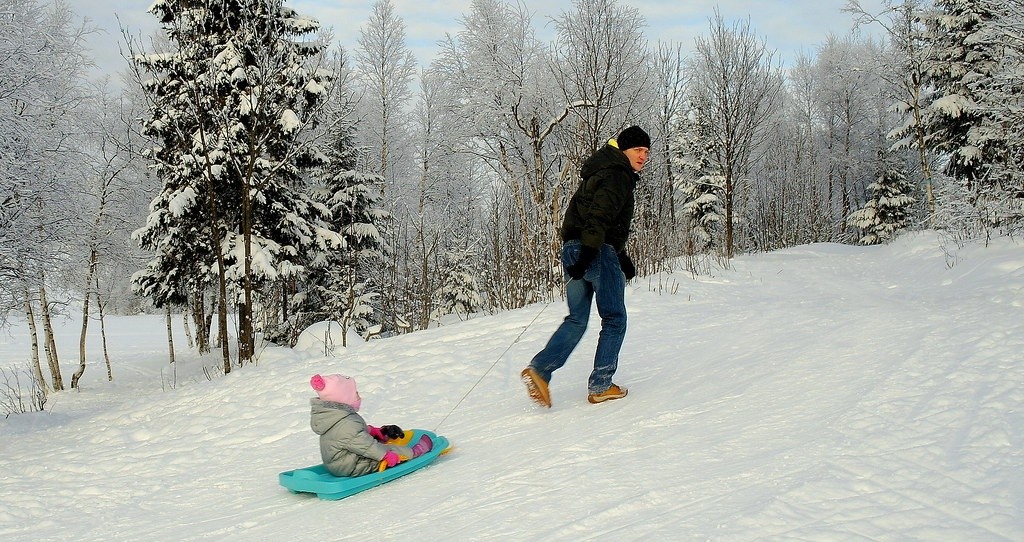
[351,398,361,412]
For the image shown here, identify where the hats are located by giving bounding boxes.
[310,374,358,405]
[616,126,650,151]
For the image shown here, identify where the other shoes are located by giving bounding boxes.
[412,434,432,458]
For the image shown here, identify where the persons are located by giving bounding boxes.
[310,373,433,478]
[521,125,651,409]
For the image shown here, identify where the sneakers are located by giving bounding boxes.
[521,368,552,409]
[588,383,628,403]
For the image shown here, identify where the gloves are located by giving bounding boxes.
[618,250,635,279]
[368,425,389,442]
[567,245,598,280]
[383,450,400,466]
